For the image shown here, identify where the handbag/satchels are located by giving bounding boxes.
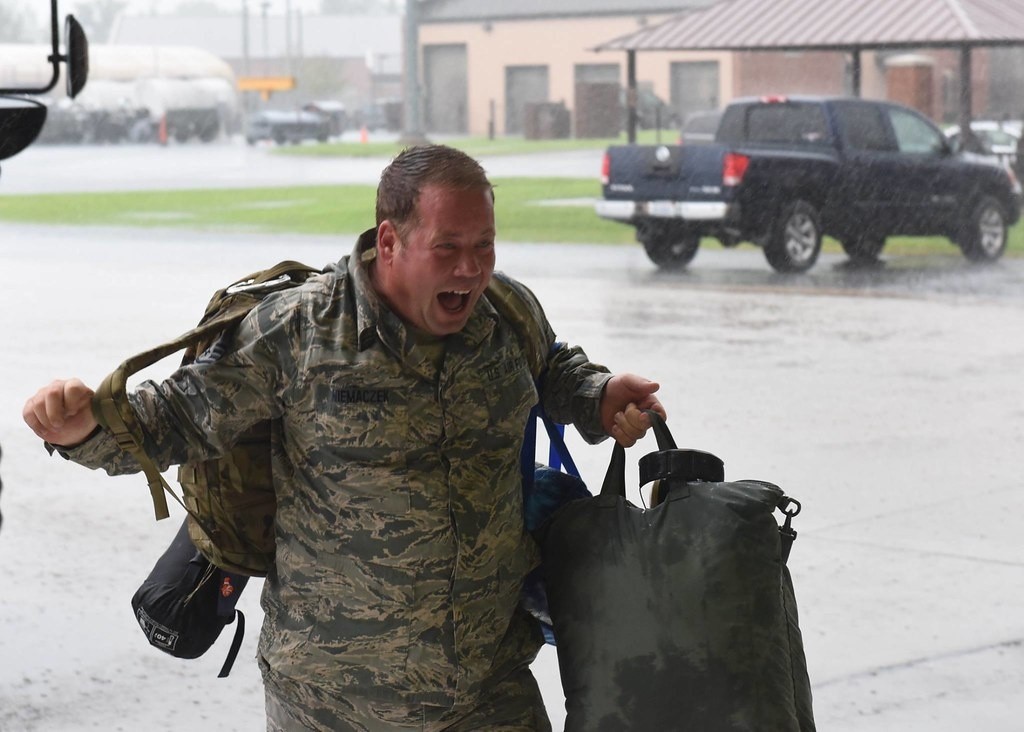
[536,409,816,732]
[520,342,593,646]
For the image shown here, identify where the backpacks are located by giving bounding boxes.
[89,260,323,578]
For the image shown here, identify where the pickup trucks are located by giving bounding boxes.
[595,97,1023,277]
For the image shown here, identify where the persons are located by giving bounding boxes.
[23,142,667,730]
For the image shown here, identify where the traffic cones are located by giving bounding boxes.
[159,119,167,146]
[360,127,368,144]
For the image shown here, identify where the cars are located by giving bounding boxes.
[161,107,219,144]
[244,111,327,146]
